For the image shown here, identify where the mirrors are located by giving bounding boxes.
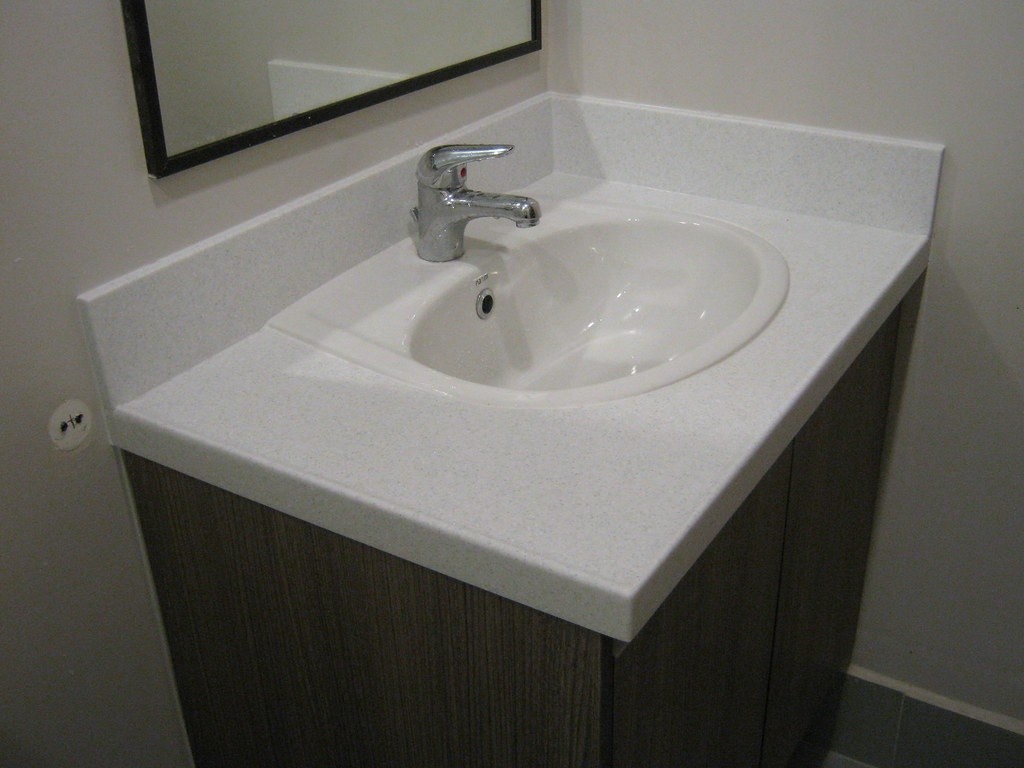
[120,0,543,180]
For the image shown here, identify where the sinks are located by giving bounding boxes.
[407,213,791,401]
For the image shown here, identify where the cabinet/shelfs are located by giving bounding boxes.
[114,266,928,767]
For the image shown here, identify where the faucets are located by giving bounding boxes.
[408,143,543,263]
